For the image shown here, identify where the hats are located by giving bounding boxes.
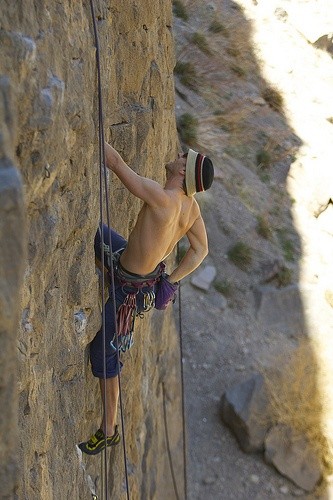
[186,149,214,197]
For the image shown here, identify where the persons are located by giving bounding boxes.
[78,140,214,455]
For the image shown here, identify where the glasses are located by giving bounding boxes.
[182,153,187,160]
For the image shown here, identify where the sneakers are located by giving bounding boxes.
[79,425,122,455]
